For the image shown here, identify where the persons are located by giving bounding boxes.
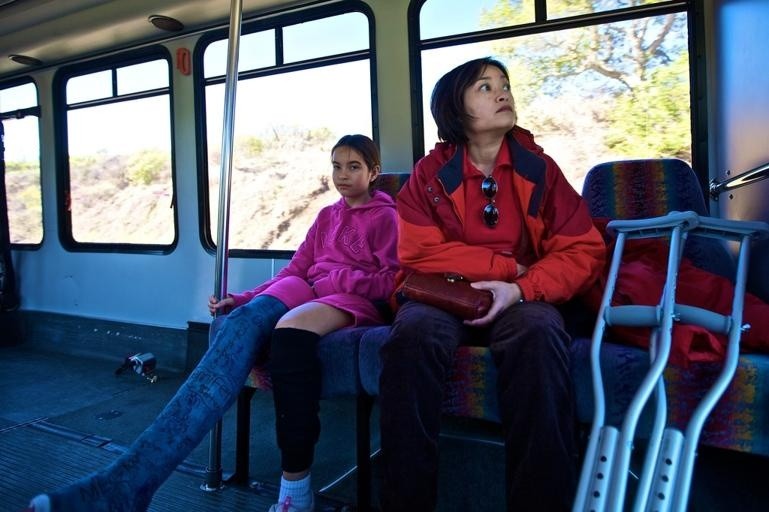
[27,134,401,512]
[379,55,608,512]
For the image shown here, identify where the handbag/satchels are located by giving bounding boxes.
[400,267,490,319]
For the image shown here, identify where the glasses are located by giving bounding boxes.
[481,174,499,230]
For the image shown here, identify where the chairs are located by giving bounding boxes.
[209,158,412,511]
[574,157,769,512]
[358,325,571,430]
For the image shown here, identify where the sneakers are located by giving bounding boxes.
[270,492,318,511]
